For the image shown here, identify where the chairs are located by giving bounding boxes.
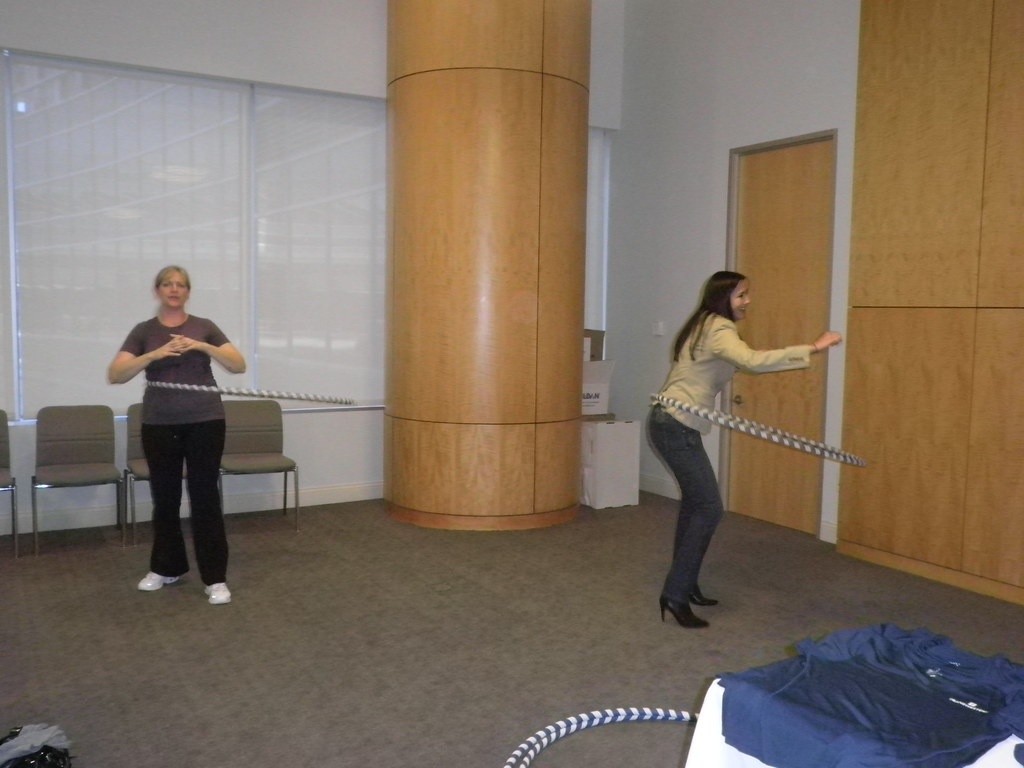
[124,404,188,547]
[0,410,19,558]
[219,400,300,535]
[31,405,126,557]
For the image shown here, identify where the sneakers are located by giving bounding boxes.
[203,582,233,605]
[137,572,178,591]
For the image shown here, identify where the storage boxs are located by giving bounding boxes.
[581,328,640,510]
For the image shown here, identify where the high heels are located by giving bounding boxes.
[659,593,709,628]
[689,587,718,606]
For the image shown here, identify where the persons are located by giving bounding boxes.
[644,269,843,630]
[107,266,245,606]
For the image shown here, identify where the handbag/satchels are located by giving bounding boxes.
[0,727,72,768]
